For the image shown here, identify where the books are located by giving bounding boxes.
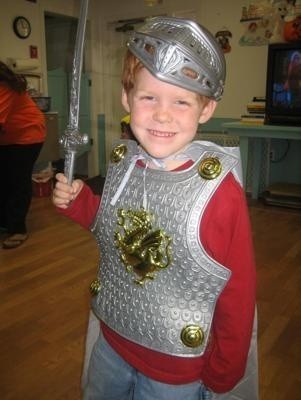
[241,94,267,126]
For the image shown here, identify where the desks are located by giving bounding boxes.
[224,123,301,200]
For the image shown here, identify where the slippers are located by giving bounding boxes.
[3,232,29,248]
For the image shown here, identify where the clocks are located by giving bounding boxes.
[14,15,32,40]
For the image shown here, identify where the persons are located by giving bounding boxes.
[53,16,259,399]
[0,59,49,250]
